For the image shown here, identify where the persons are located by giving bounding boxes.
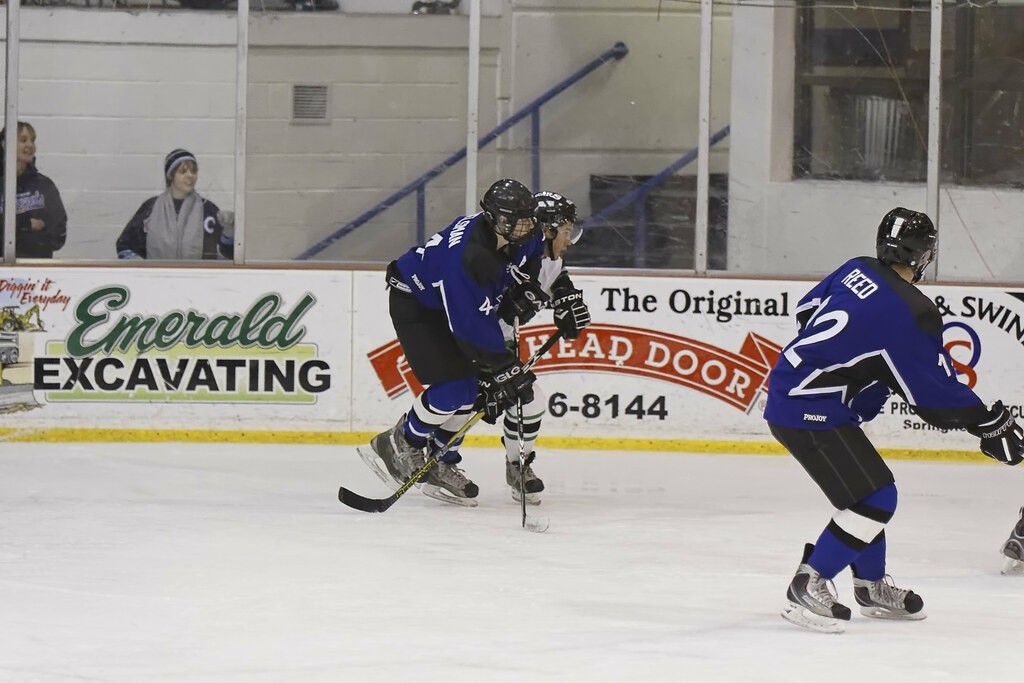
[357,178,538,507]
[0,122,67,258]
[115,148,234,259]
[471,191,592,505]
[763,208,1024,634]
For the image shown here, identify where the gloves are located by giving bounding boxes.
[553,288,591,339]
[967,399,1024,466]
[471,381,509,425]
[217,211,234,236]
[492,352,536,404]
[501,282,551,327]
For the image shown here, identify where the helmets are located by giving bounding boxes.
[480,179,537,245]
[876,206,940,283]
[531,191,585,245]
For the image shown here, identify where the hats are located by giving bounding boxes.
[165,148,196,180]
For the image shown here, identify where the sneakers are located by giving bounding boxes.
[787,543,851,620]
[852,575,924,614]
[1000,506,1023,561]
[501,436,544,492]
[370,413,426,485]
[426,437,479,497]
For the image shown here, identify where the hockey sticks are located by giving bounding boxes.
[336,327,566,515]
[511,314,552,533]
